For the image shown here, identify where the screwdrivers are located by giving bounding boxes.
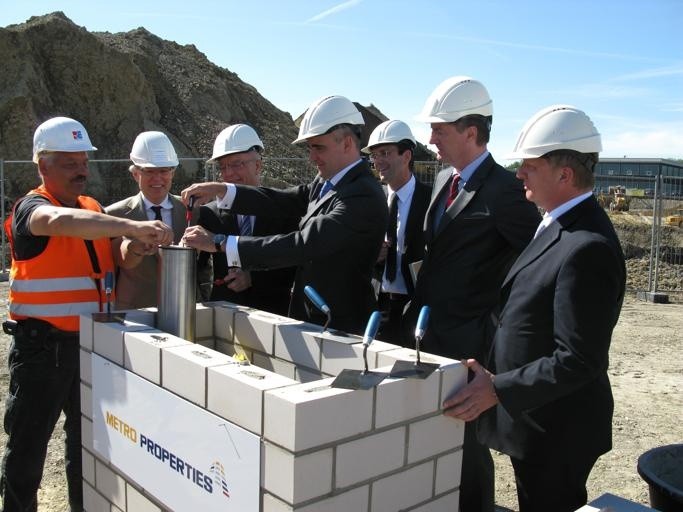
[186,196,194,227]
[200,279,232,286]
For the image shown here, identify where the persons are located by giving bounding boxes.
[202,122,296,317]
[0,114,172,512]
[442,105,628,511]
[103,131,215,309]
[410,78,543,511]
[361,120,433,318]
[176,96,389,339]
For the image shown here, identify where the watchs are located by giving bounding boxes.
[215,233,224,249]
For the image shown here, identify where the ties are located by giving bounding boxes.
[386,194,400,284]
[448,174,461,208]
[320,179,334,198]
[238,215,252,237]
[150,206,163,222]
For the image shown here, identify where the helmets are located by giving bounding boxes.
[416,75,493,123]
[32,117,98,164]
[205,123,264,163]
[506,104,602,161]
[361,119,417,153]
[130,131,180,169]
[290,95,365,145]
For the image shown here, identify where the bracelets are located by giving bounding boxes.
[130,249,143,259]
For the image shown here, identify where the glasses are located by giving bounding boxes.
[370,150,390,163]
